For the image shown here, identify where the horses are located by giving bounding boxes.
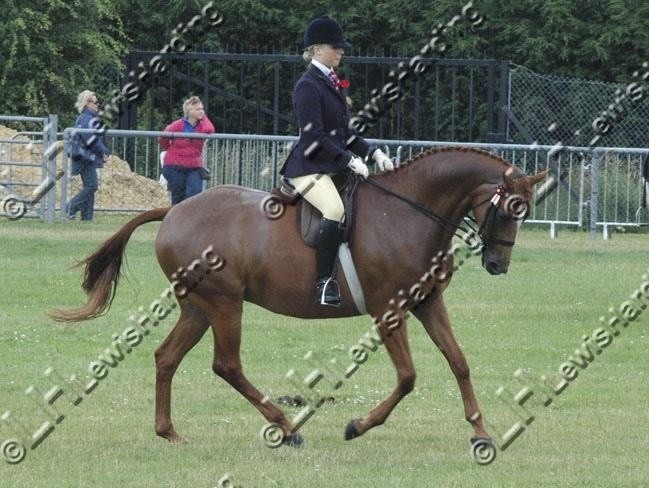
[44,144,552,452]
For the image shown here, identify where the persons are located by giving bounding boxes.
[158,95,216,204]
[280,15,394,303]
[64,89,112,221]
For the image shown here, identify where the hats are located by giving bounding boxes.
[302,15,353,49]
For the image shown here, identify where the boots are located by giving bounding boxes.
[315,217,344,303]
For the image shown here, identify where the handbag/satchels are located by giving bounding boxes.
[72,132,104,169]
[200,169,210,180]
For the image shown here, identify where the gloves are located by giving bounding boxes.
[346,156,369,180]
[372,149,394,172]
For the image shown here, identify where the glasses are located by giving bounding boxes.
[89,100,99,105]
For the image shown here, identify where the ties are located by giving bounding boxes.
[331,72,341,92]
